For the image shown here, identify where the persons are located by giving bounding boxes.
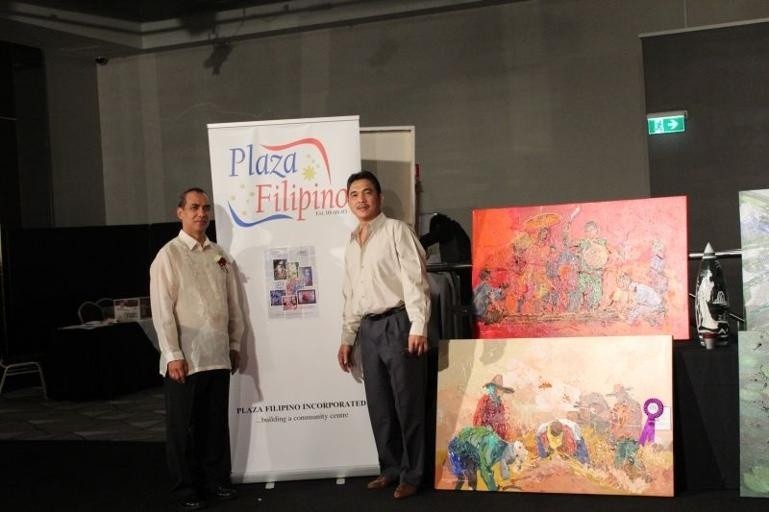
[446,372,643,492]
[144,185,247,509]
[335,169,436,501]
[471,219,674,330]
[419,212,473,325]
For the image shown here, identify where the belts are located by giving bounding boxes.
[361,304,405,322]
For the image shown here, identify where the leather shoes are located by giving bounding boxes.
[178,496,202,509]
[367,474,398,491]
[393,481,419,498]
[212,485,237,498]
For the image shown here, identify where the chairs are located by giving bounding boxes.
[1,352,53,404]
[55,296,158,398]
